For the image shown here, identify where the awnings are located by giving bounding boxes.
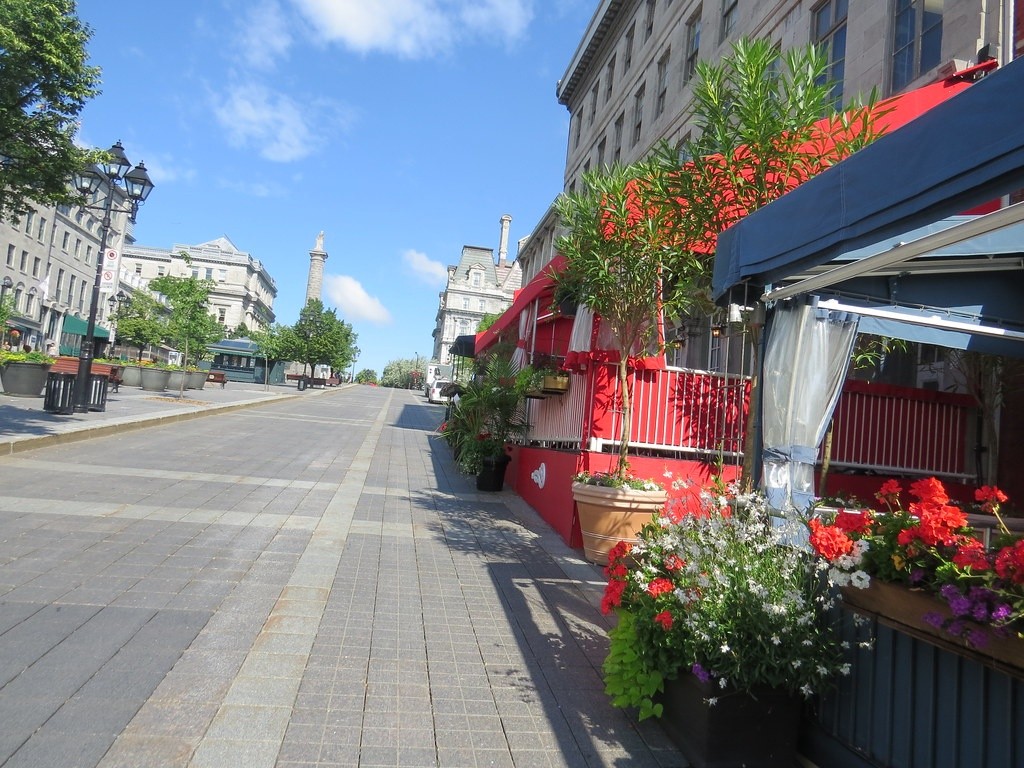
[63,315,110,337]
[449,334,475,358]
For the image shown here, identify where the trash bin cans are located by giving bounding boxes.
[43,371,78,415]
[88,372,109,412]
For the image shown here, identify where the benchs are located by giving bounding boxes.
[287,374,309,381]
[206,371,227,389]
[326,379,339,386]
[49,356,125,393]
[308,378,326,388]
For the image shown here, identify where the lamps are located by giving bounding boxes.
[672,335,684,350]
[710,321,724,338]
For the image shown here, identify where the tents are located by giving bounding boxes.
[715,58,1024,522]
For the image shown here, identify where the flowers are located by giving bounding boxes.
[600,465,872,725]
[805,477,1022,655]
[542,347,570,383]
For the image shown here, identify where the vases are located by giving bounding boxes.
[839,568,1022,681]
[543,376,569,391]
[649,663,818,766]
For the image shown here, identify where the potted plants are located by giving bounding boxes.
[93,357,209,393]
[430,343,534,492]
[539,140,705,567]
[0,351,57,398]
